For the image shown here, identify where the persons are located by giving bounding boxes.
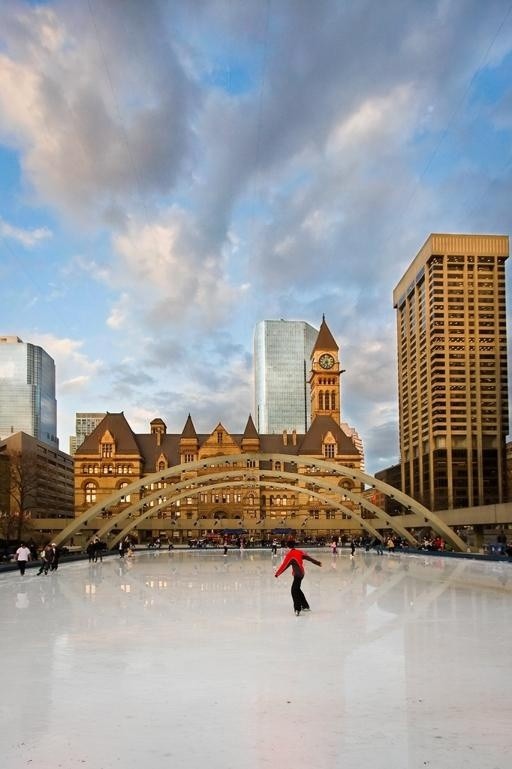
[331,534,408,556]
[236,535,255,548]
[261,537,285,555]
[86,534,104,563]
[299,537,326,547]
[271,537,322,616]
[147,534,161,550]
[15,538,39,576]
[188,534,221,549]
[223,540,228,554]
[37,543,58,575]
[419,535,441,553]
[119,534,135,559]
[167,537,174,551]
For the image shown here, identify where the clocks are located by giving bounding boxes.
[319,354,335,369]
